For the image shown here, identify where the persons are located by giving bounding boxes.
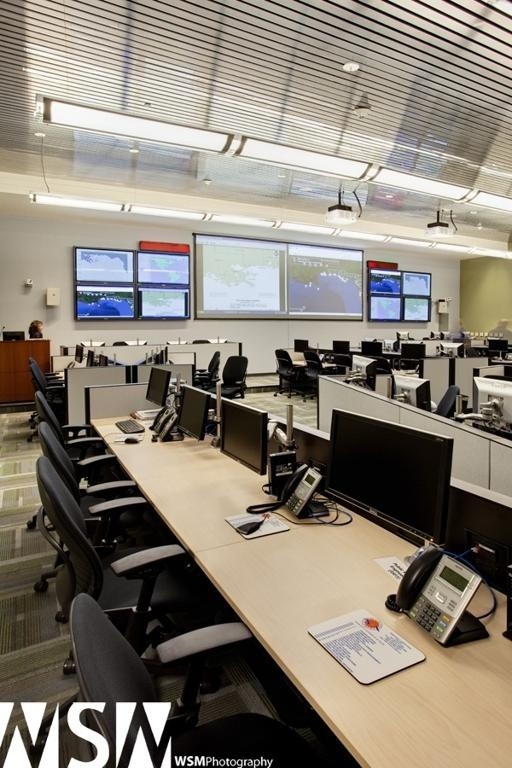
[28,320,44,338]
[484,318,512,344]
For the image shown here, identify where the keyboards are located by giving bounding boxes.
[115,420,145,433]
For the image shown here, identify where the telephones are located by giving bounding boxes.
[281,464,329,520]
[394,549,489,648]
[153,406,184,442]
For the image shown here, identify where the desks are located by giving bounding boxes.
[0,339,52,403]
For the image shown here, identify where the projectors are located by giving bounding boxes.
[323,209,357,225]
[426,226,454,238]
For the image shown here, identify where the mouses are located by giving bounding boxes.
[125,438,139,443]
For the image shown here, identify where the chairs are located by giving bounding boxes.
[24,325,512,768]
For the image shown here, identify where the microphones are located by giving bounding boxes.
[0,326,5,340]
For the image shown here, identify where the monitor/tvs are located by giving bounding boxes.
[220,397,268,475]
[294,331,509,357]
[367,268,432,322]
[352,355,378,388]
[177,387,211,440]
[2,331,25,341]
[74,339,229,366]
[74,246,192,320]
[145,367,171,406]
[473,377,512,424]
[324,408,454,546]
[394,375,431,412]
